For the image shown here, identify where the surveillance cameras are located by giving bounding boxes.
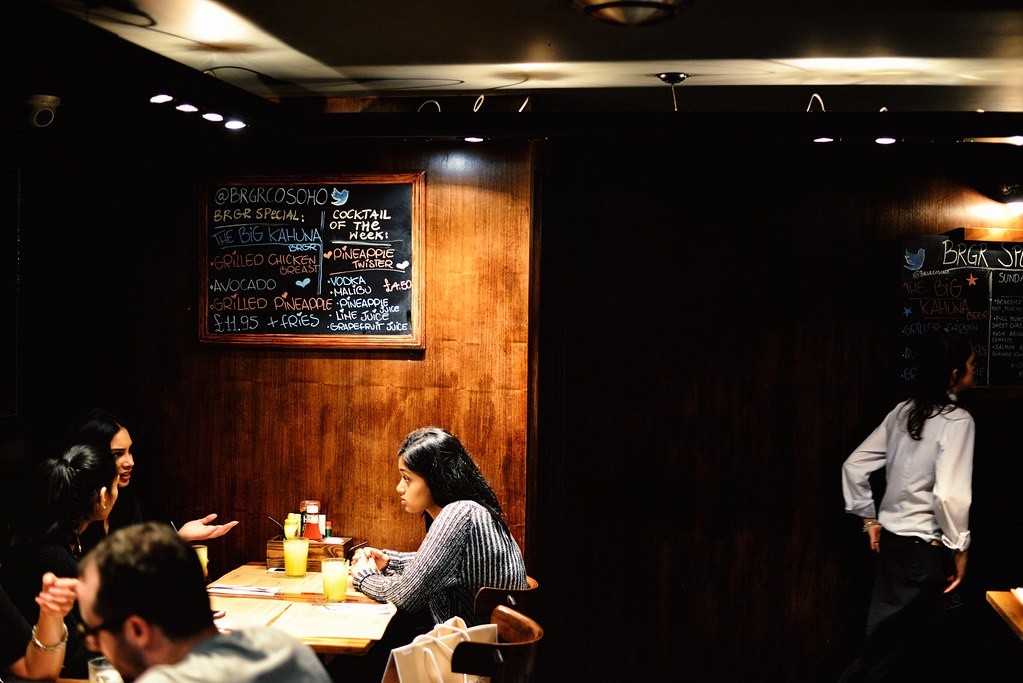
[23,95,61,127]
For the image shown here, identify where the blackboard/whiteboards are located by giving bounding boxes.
[889,227,1023,401]
[197,170,426,351]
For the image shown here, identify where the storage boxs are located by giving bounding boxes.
[264,535,352,572]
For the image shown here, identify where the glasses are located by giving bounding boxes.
[77,623,115,651]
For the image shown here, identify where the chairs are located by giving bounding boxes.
[450,604,543,683]
[473,574,539,614]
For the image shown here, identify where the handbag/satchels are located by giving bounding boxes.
[381,616,498,683]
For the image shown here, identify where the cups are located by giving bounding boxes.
[191,544,208,577]
[88,657,125,683]
[283,537,310,577]
[320,557,349,603]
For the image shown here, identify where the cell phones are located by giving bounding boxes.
[268,568,285,574]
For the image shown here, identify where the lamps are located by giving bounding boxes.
[560,0,696,29]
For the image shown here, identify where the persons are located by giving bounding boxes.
[0,444,122,679]
[0,572,80,683]
[839,329,974,683]
[77,409,240,543]
[78,522,331,683]
[349,425,528,627]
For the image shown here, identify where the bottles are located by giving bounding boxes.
[326,522,331,537]
[302,505,322,542]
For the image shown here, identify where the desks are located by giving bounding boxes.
[985,590,1023,640]
[207,561,382,656]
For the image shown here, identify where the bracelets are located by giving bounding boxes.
[863,520,876,532]
[32,624,69,652]
